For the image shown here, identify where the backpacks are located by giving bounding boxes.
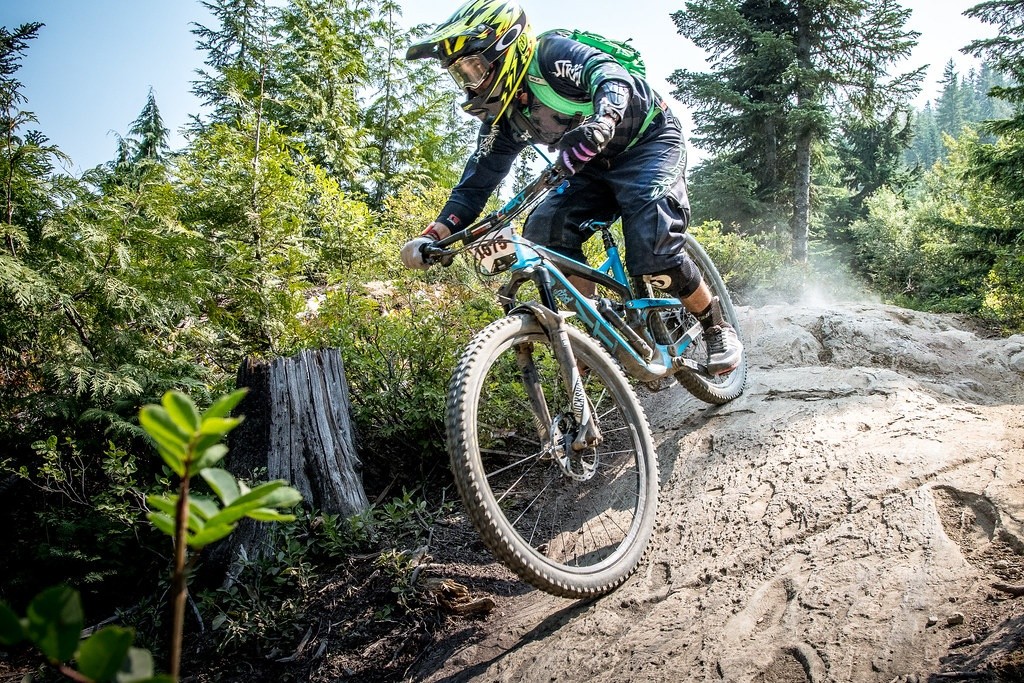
[527,29,646,116]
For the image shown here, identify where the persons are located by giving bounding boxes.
[399,0,744,374]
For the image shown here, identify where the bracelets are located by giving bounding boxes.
[421,222,442,241]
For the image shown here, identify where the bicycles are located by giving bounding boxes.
[414,128,749,599]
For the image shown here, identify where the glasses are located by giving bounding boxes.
[447,11,527,94]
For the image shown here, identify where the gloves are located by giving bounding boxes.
[399,235,435,271]
[559,114,615,176]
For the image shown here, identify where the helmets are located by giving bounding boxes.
[407,0,536,128]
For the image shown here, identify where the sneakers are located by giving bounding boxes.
[701,321,743,376]
